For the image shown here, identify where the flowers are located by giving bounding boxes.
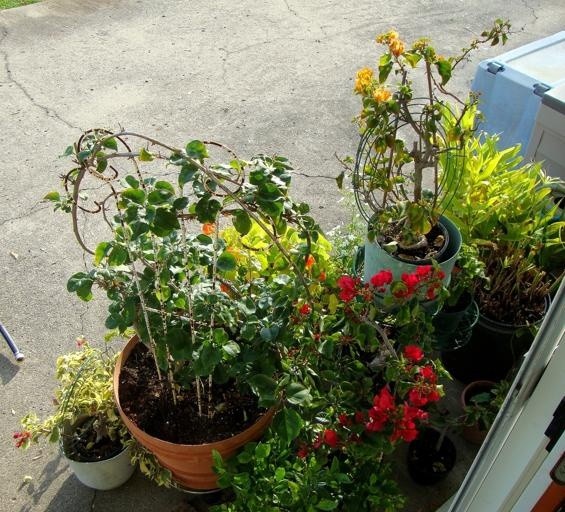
[348,19,525,239]
[13,325,135,452]
[198,211,452,462]
[348,19,526,134]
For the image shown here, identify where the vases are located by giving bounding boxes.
[63,415,136,490]
[113,326,284,493]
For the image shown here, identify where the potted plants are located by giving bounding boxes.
[349,134,565,512]
